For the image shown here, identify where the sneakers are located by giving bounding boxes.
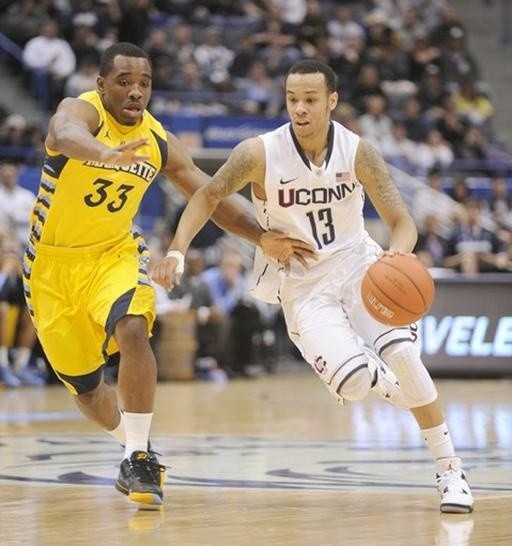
[436,457,474,513]
[0,363,46,388]
[114,441,166,510]
[196,357,271,383]
[360,346,410,410]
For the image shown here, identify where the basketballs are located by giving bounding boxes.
[362,253,435,326]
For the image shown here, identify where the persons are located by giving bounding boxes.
[19,40,321,511]
[147,55,482,516]
